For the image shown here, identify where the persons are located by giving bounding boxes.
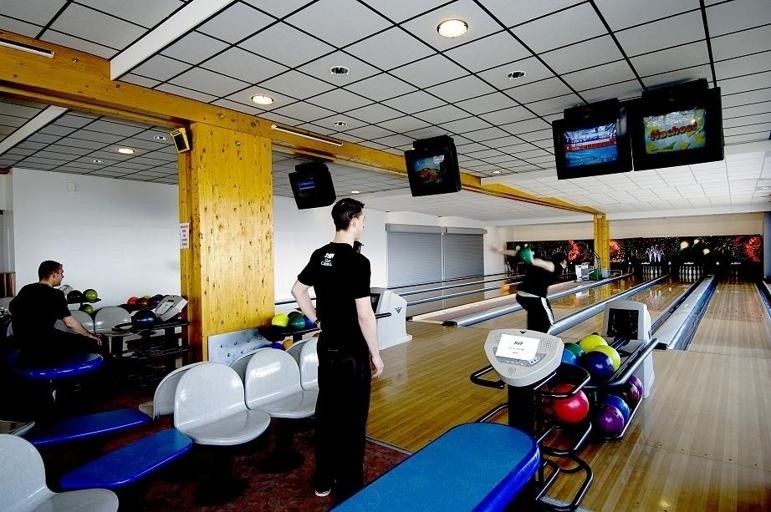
[9,260,115,360]
[289,198,386,505]
[490,242,568,334]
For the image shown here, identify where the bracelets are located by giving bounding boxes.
[312,319,321,331]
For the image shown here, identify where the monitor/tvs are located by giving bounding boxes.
[287,161,336,210]
[625,77,726,171]
[404,134,462,198]
[550,97,632,179]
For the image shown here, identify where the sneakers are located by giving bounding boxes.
[314,472,335,498]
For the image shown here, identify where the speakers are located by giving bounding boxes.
[170,128,191,155]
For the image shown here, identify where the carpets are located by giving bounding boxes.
[74,390,589,512]
